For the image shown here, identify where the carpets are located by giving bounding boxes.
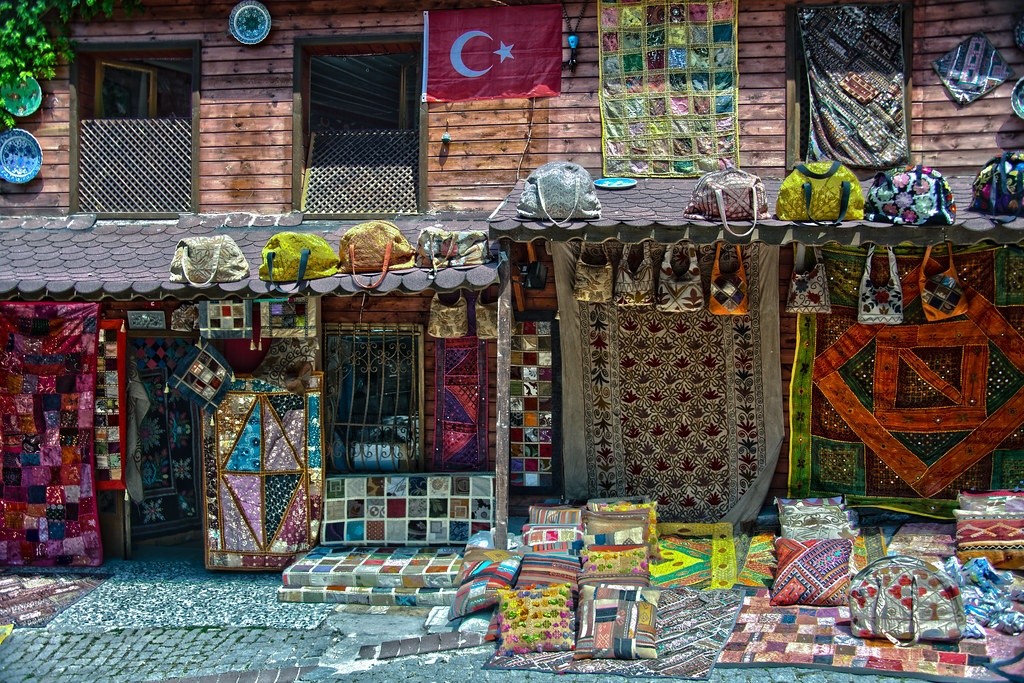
[648,522,739,591]
[322,476,497,546]
[712,584,1024,683]
[0,303,325,630]
[434,332,489,471]
[596,0,738,178]
[788,241,1024,521]
[884,519,956,559]
[550,239,786,532]
[931,29,1015,106]
[739,527,889,588]
[795,3,910,170]
[482,586,747,681]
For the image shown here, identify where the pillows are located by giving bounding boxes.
[953,488,1024,572]
[770,495,860,607]
[447,494,661,661]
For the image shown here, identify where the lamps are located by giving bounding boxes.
[516,259,547,292]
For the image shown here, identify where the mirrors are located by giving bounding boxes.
[95,59,158,120]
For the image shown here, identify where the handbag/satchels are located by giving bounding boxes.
[572,242,968,326]
[683,168,771,236]
[864,163,957,226]
[517,162,603,226]
[476,288,518,342]
[258,231,338,294]
[966,150,1024,224]
[170,235,250,287]
[339,220,416,289]
[416,225,492,279]
[846,555,967,646]
[777,161,862,227]
[428,294,470,339]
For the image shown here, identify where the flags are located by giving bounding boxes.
[420,9,561,102]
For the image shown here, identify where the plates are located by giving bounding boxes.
[0,129,44,184]
[594,178,638,189]
[229,0,272,45]
[1011,77,1024,120]
[0,76,42,117]
